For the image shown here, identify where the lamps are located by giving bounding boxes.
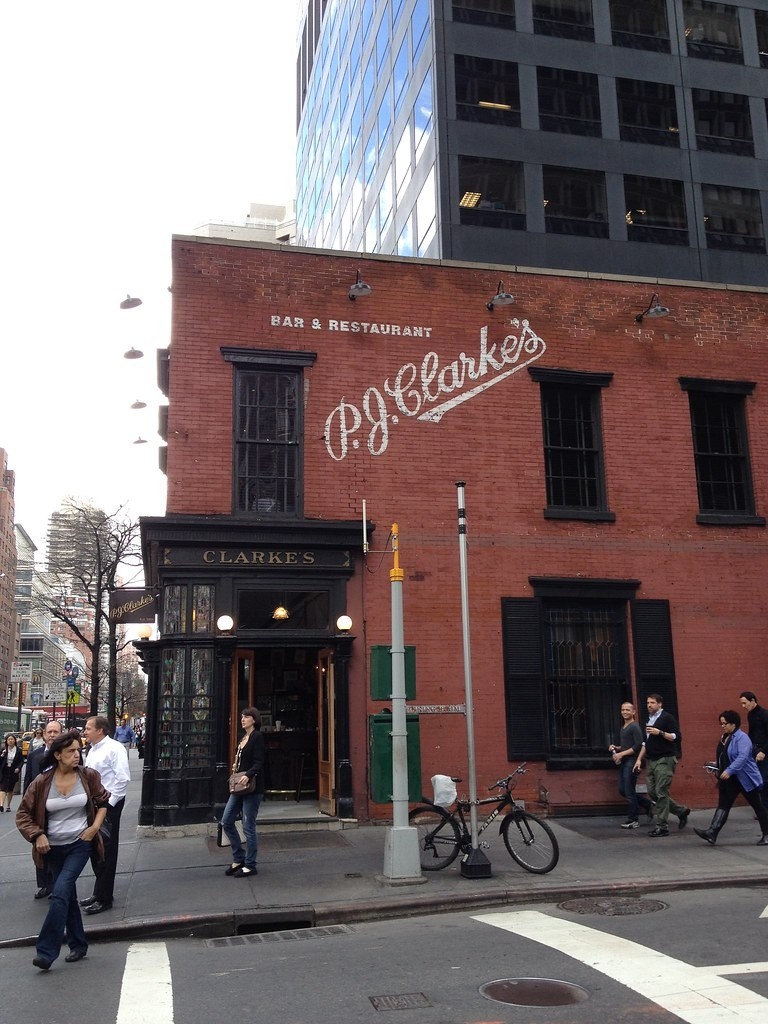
[487,280,514,310]
[636,294,670,322]
[349,269,372,300]
[217,615,234,636]
[272,607,289,619]
[336,615,352,634]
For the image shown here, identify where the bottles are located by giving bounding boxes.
[611,748,621,765]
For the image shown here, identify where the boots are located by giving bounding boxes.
[756,816,768,846]
[694,809,729,845]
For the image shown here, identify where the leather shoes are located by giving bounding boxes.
[647,828,670,838]
[80,896,112,913]
[32,956,46,969]
[678,808,690,829]
[65,949,86,963]
[34,888,54,899]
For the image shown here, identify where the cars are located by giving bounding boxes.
[21,732,34,758]
[32,721,45,731]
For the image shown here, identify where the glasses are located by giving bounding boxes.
[719,722,729,725]
[36,732,43,734]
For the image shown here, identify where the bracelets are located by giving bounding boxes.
[659,730,665,736]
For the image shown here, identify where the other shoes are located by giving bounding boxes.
[0,806,4,812]
[225,861,257,878]
[6,808,11,812]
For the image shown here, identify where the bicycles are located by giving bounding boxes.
[409,761,559,875]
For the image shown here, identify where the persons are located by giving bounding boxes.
[220,706,270,877]
[692,711,768,846]
[77,715,130,913]
[15,732,112,968]
[0,719,146,813]
[608,702,656,829]
[23,721,84,900]
[632,694,691,837]
[740,691,768,819]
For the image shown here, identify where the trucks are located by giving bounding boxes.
[0,705,32,751]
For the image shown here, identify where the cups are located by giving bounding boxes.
[276,721,281,732]
[646,723,654,727]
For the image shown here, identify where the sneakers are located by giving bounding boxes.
[647,802,657,824]
[621,819,640,829]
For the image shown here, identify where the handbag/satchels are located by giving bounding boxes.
[702,760,719,773]
[229,771,256,794]
[217,811,246,847]
